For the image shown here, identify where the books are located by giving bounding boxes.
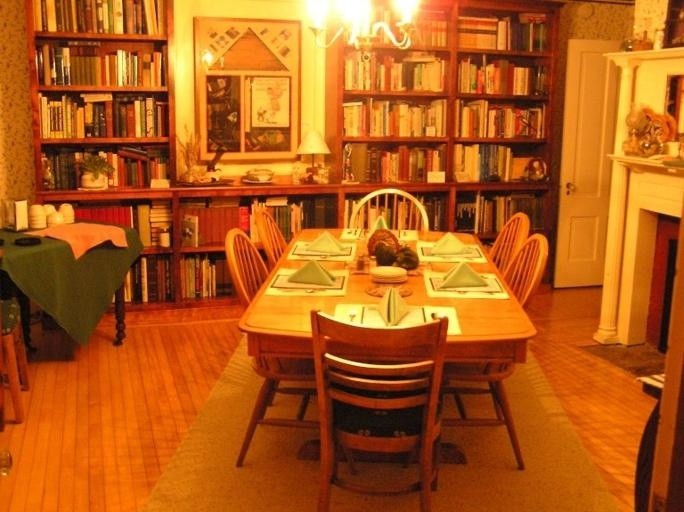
[455,99,545,138]
[343,144,447,183]
[455,191,545,233]
[345,195,448,232]
[180,254,234,297]
[43,146,167,189]
[113,255,173,304]
[181,197,336,247]
[343,97,446,137]
[346,7,447,46]
[454,144,546,182]
[33,0,163,35]
[345,51,445,91]
[39,93,167,138]
[458,55,546,94]
[457,12,548,52]
[39,41,167,86]
[76,201,172,247]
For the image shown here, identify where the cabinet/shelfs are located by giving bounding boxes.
[179,191,336,305]
[334,0,553,277]
[27,0,174,310]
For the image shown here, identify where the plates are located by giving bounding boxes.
[369,266,407,283]
[240,179,273,183]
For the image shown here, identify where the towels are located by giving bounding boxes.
[442,263,490,288]
[23,220,129,258]
[431,232,470,255]
[379,287,409,326]
[304,233,346,254]
[288,258,335,286]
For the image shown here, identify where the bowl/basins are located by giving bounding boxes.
[58,203,74,223]
[46,212,63,228]
[245,169,274,181]
[41,205,56,215]
[28,204,46,228]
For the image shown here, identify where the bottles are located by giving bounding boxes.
[358,255,363,270]
[368,255,376,271]
[653,28,664,51]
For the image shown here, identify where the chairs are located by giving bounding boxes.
[311,311,448,512]
[226,226,358,467]
[0,297,30,426]
[255,209,289,263]
[348,189,429,232]
[485,212,527,278]
[424,234,549,472]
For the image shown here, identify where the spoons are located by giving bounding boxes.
[346,228,352,233]
[282,290,314,293]
[348,312,355,322]
[298,255,327,258]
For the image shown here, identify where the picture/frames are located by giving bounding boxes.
[193,17,303,162]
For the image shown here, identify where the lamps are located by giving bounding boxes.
[299,0,425,46]
